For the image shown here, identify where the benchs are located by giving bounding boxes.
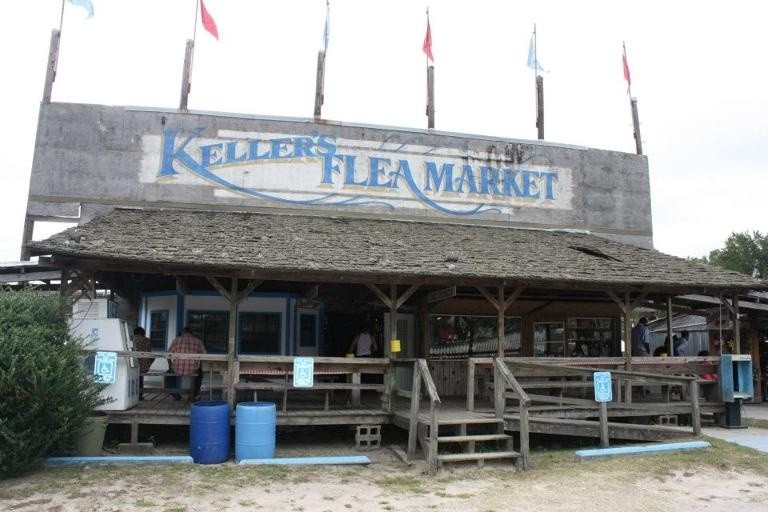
[140,373,199,403]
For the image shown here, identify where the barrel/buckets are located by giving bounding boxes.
[234,402,276,461]
[75,410,109,456]
[189,401,231,464]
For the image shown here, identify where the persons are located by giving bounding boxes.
[652,335,669,356]
[236,336,262,400]
[630,316,654,395]
[695,350,719,393]
[131,326,155,402]
[166,326,207,402]
[348,325,378,401]
[672,329,690,358]
[672,334,681,342]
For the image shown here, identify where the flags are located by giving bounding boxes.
[620,45,632,92]
[66,0,95,16]
[526,32,545,72]
[197,0,219,41]
[420,11,433,64]
[318,3,330,52]
